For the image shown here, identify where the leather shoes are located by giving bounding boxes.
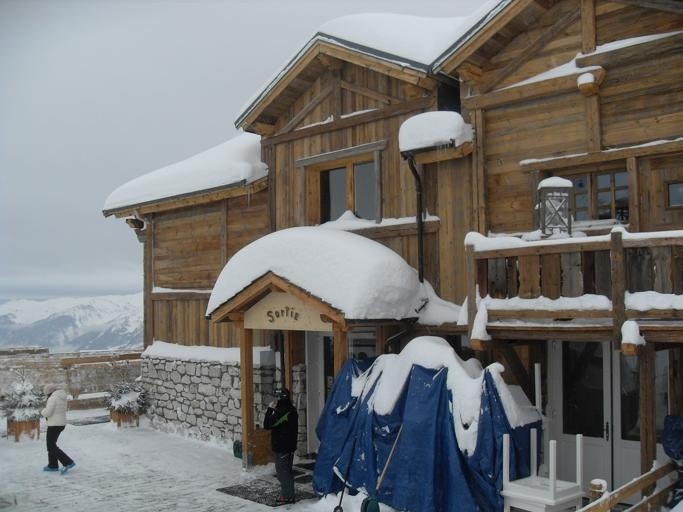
[269,401,276,408]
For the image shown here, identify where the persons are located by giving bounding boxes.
[39,383,76,474]
[263,388,298,502]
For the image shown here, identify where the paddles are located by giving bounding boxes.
[214,478,316,507]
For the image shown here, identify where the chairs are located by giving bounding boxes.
[276,496,295,504]
[44,461,76,474]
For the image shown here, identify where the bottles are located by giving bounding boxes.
[43,383,57,395]
[276,387,290,398]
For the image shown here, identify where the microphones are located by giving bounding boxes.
[499,427,586,512]
